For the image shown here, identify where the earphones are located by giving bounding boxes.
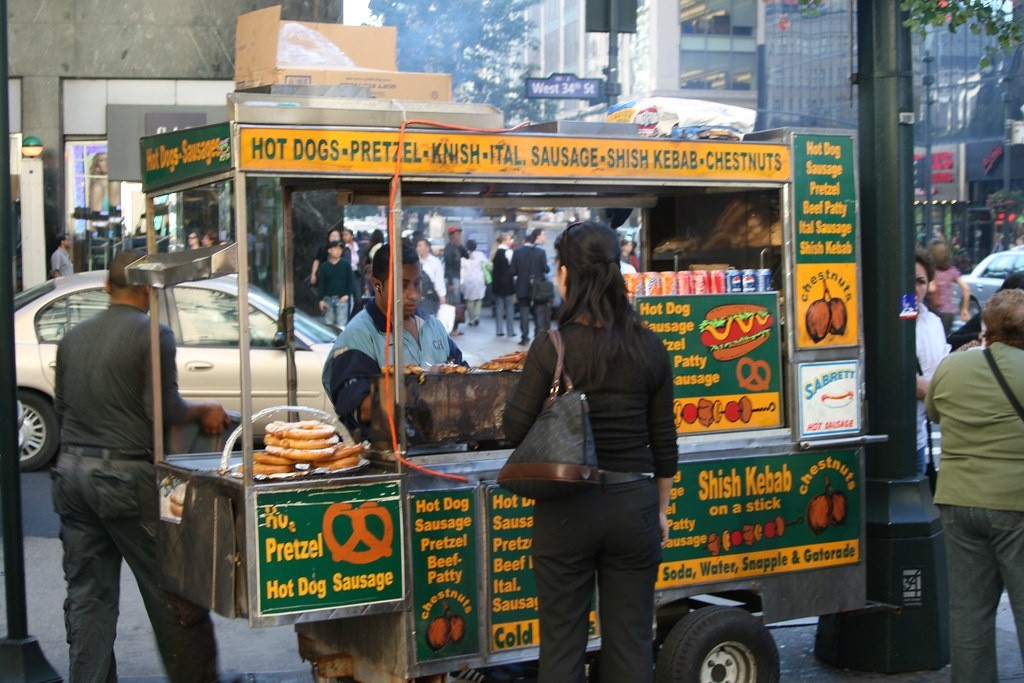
[375,283,383,295]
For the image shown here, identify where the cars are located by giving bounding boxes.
[960,245,1024,320]
[14,269,344,472]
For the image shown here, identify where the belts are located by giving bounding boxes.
[60,444,152,461]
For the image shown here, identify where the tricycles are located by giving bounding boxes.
[125,117,889,683]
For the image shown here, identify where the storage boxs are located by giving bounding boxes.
[234,4,453,101]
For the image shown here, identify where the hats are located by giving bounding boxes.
[327,240,345,247]
[447,225,462,236]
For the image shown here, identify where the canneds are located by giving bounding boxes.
[622,269,773,296]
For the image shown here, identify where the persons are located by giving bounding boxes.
[52,250,256,683]
[618,238,639,275]
[85,152,107,239]
[322,242,470,457]
[49,232,74,278]
[308,222,555,346]
[913,222,1024,495]
[186,229,219,250]
[925,289,1024,683]
[502,220,680,683]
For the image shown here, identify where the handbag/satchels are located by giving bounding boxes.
[480,259,494,285]
[497,320,606,499]
[448,290,466,323]
[533,277,555,302]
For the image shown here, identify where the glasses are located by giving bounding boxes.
[554,222,584,264]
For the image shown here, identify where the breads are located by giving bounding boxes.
[238,421,364,475]
[170,482,186,518]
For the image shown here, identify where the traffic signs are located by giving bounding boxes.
[523,73,603,101]
